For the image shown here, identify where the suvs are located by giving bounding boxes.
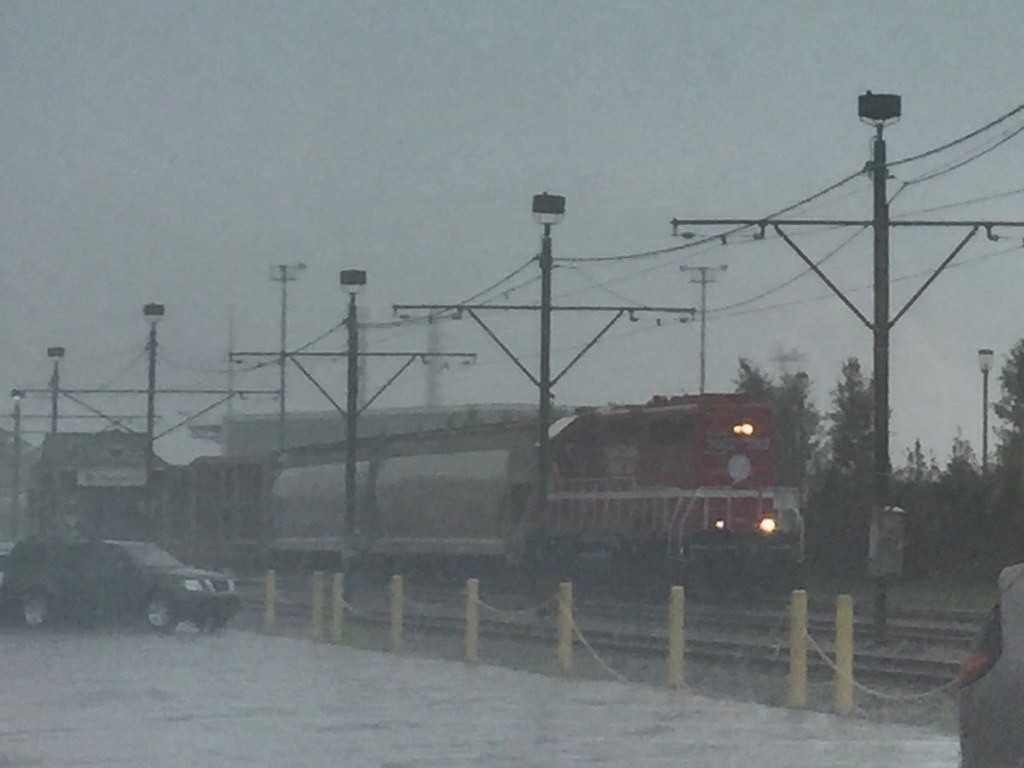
[4,536,237,637]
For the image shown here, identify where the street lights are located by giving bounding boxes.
[855,92,901,646]
[143,302,165,529]
[340,269,367,584]
[269,262,301,446]
[46,345,64,521]
[979,349,994,564]
[9,389,24,526]
[533,192,563,591]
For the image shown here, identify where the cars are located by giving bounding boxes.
[953,565,1022,768]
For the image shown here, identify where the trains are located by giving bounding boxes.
[140,393,801,602]
